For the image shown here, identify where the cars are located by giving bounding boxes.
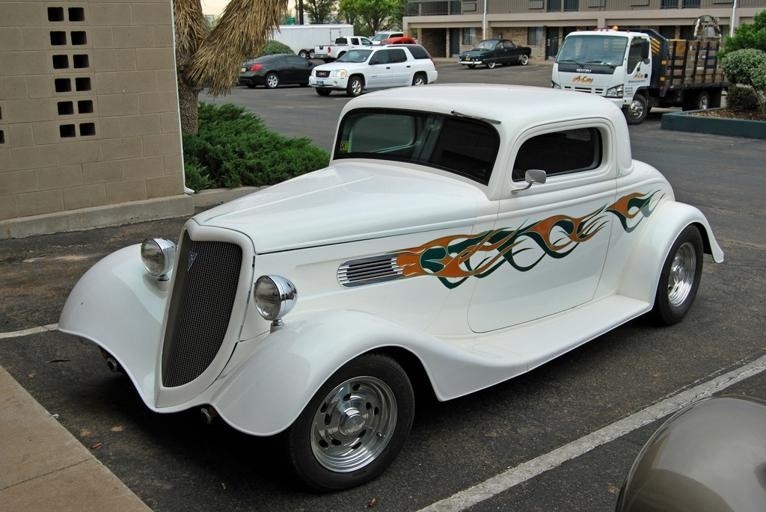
[56,84,728,495]
[239,54,326,89]
[370,31,418,46]
[458,39,532,69]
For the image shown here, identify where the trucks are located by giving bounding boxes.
[550,28,727,126]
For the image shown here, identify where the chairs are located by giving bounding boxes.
[485,136,561,186]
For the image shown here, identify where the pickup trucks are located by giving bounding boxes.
[314,36,375,63]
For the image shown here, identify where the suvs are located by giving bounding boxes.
[308,47,439,96]
